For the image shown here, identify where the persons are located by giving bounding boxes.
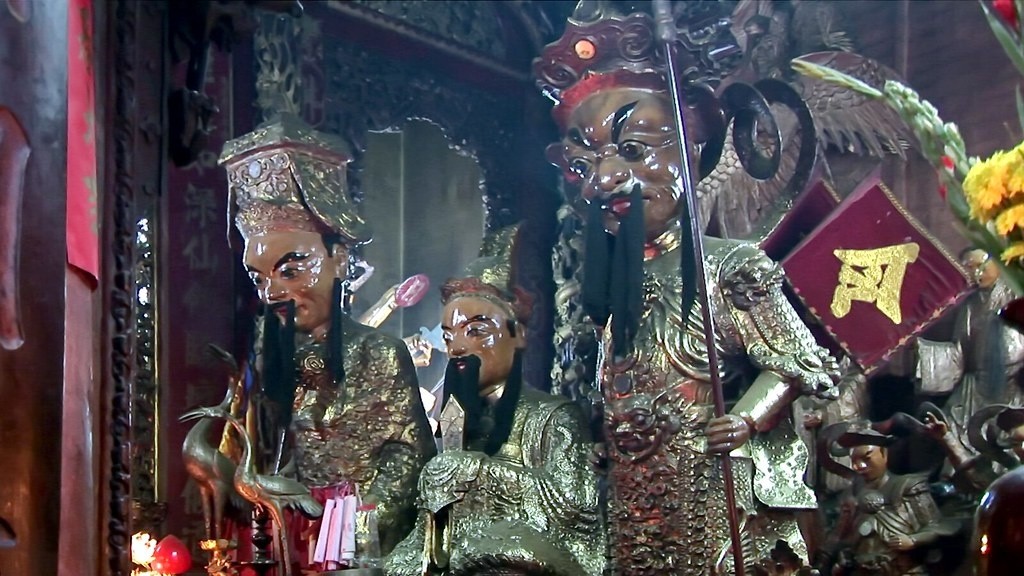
[379,224,614,576]
[803,248,1024,576]
[219,115,437,575]
[530,0,842,576]
